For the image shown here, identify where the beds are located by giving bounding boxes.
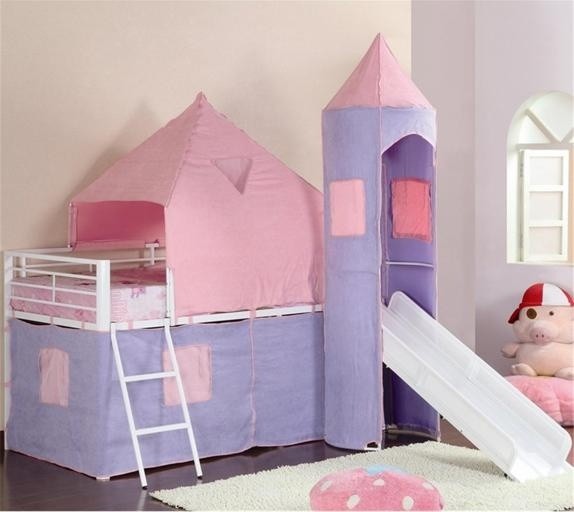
[1,240,324,488]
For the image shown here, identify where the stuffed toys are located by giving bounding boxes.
[498,283,573,379]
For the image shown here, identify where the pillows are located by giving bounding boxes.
[309,467,443,511]
[505,375,574,427]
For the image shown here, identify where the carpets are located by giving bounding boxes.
[149,440,574,510]
